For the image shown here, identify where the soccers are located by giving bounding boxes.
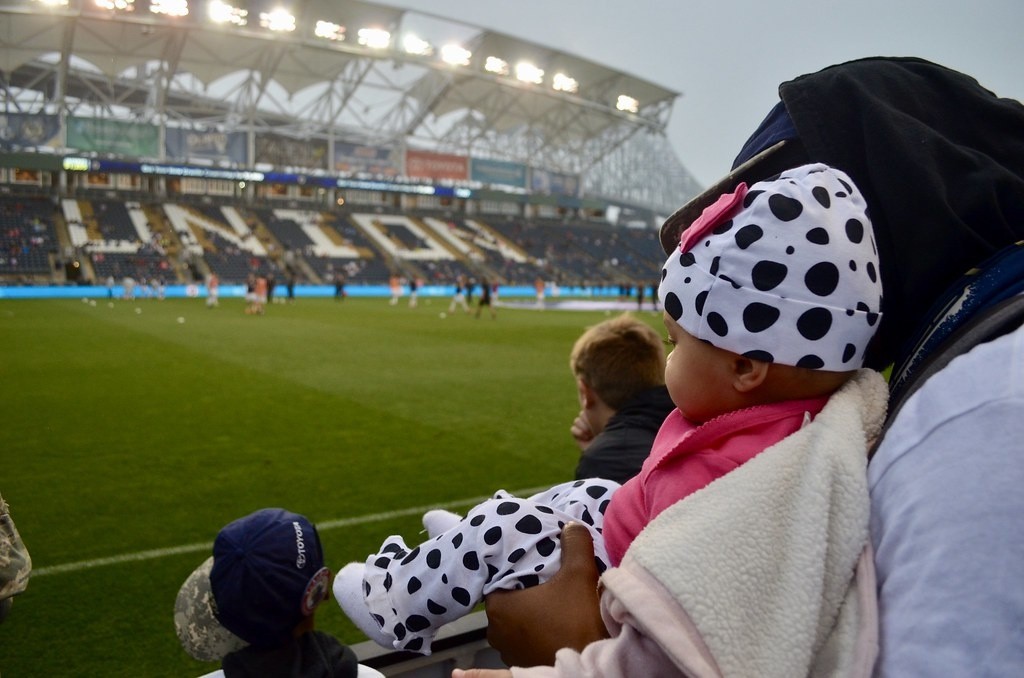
[82,298,447,324]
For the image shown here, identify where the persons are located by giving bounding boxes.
[2,188,662,313]
[570,316,675,484]
[173,508,385,678]
[487,58,1024,676]
[332,163,889,678]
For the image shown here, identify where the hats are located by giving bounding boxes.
[657,100,810,259]
[172,508,330,662]
[656,163,884,372]
